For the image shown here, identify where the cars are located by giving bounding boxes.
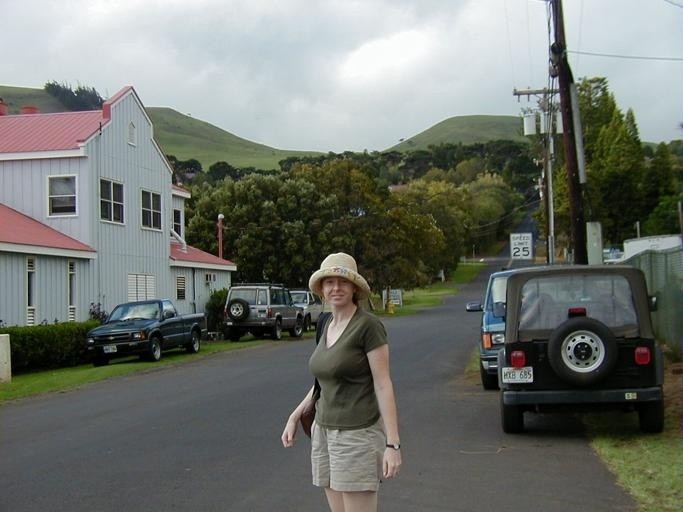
[289,290,323,331]
[466,266,517,390]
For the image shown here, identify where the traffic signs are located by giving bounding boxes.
[510,232,534,261]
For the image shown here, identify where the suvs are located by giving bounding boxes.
[491,264,664,433]
[223,283,306,342]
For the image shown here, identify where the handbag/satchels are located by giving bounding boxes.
[301,392,322,438]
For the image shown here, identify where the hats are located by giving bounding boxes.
[308,253,371,300]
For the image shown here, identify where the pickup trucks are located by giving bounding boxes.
[86,298,208,366]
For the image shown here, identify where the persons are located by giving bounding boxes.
[281,252,403,512]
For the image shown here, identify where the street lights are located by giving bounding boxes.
[513,87,564,264]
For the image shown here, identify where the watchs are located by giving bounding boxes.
[385,444,401,450]
